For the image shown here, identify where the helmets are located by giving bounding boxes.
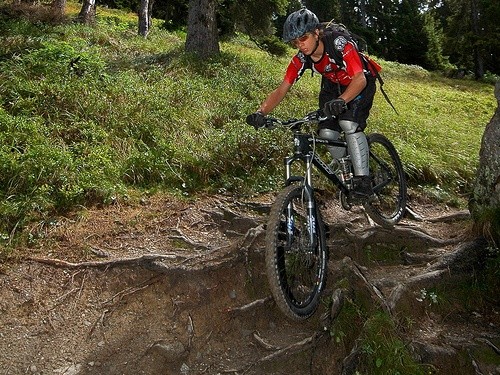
[282,9,319,44]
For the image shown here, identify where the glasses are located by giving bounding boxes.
[291,32,311,45]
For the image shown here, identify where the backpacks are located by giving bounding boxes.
[296,22,369,78]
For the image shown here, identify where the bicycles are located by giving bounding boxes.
[248,104,408,321]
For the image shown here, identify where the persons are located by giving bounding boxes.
[247,7,382,198]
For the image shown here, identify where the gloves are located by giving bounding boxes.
[323,99,346,118]
[246,112,266,131]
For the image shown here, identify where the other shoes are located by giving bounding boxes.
[354,175,373,196]
